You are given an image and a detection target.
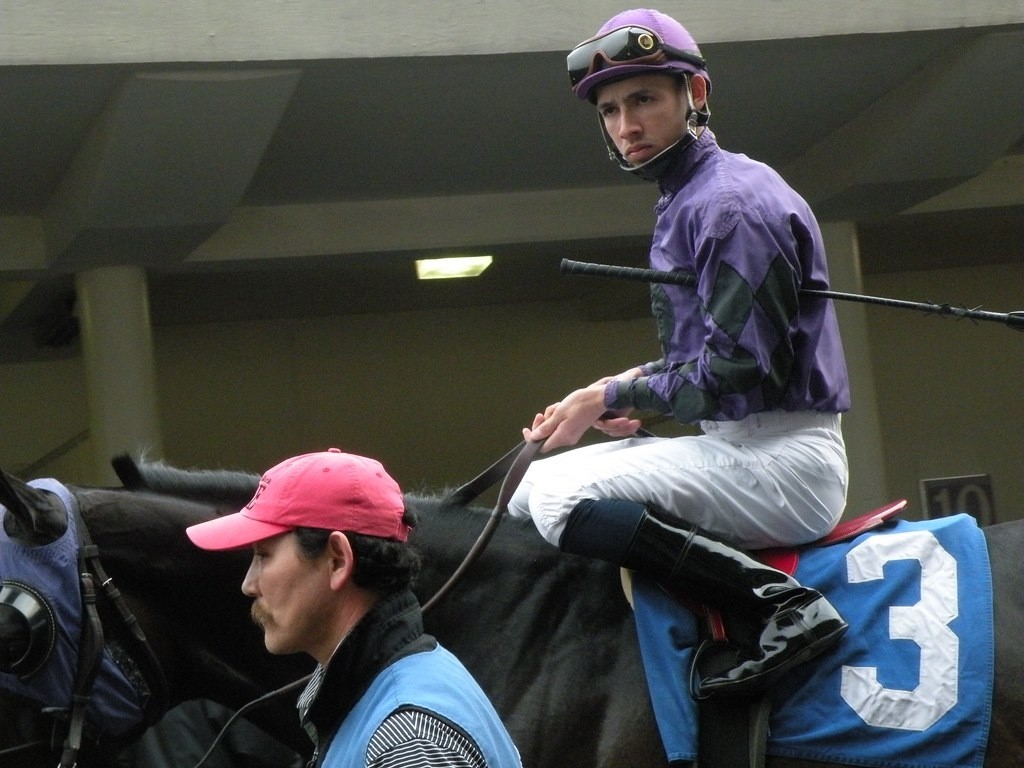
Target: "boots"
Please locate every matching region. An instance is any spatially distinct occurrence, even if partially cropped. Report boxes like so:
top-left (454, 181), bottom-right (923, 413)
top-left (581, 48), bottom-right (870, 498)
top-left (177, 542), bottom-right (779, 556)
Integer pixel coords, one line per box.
top-left (618, 501), bottom-right (849, 698)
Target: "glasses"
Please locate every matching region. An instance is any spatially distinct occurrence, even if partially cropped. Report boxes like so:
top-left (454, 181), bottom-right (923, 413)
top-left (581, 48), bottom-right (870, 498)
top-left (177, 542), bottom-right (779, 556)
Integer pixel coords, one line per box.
top-left (565, 24), bottom-right (707, 94)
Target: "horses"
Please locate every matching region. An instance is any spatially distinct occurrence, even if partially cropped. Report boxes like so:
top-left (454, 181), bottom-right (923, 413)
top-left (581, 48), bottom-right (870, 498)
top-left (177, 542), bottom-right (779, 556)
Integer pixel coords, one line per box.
top-left (0, 453), bottom-right (1024, 768)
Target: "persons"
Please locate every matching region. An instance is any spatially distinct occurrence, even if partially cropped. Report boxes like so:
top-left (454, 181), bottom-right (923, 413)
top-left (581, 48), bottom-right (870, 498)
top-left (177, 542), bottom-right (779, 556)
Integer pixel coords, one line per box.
top-left (185, 447), bottom-right (521, 768)
top-left (505, 7), bottom-right (850, 697)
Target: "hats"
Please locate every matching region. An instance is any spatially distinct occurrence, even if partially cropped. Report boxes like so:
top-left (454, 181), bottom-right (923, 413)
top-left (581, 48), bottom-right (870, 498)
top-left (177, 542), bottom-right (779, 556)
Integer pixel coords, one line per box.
top-left (186, 448), bottom-right (413, 550)
top-left (571, 7), bottom-right (713, 104)
